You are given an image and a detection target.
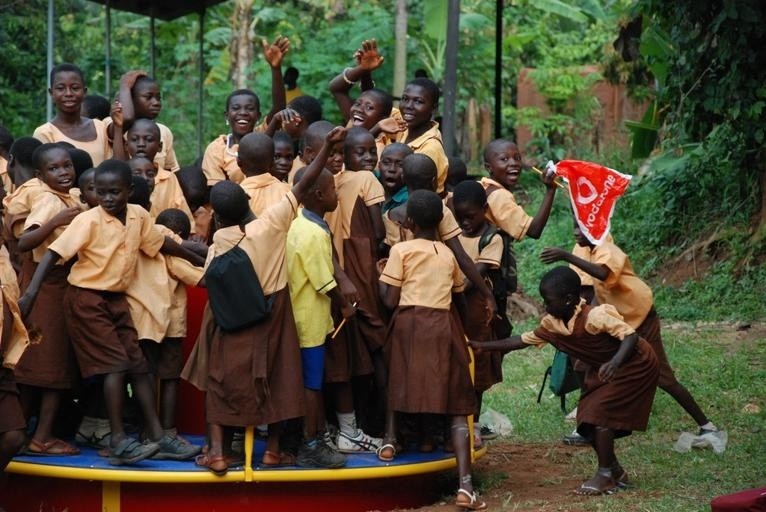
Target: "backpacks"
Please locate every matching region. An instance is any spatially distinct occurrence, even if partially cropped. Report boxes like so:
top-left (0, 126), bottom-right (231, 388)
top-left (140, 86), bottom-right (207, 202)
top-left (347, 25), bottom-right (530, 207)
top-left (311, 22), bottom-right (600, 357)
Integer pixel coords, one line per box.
top-left (536, 350), bottom-right (584, 416)
top-left (202, 235), bottom-right (276, 334)
top-left (478, 225), bottom-right (512, 333)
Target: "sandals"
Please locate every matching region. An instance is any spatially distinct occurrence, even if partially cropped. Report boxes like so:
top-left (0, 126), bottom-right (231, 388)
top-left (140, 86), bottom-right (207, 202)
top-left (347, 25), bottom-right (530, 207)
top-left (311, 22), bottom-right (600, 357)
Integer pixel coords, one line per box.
top-left (572, 467), bottom-right (628, 497)
top-left (90, 429), bottom-right (112, 448)
top-left (455, 487), bottom-right (488, 508)
top-left (375, 444), bottom-right (397, 461)
top-left (75, 432), bottom-right (97, 448)
top-left (195, 451), bottom-right (229, 474)
top-left (24, 437), bottom-right (81, 457)
top-left (258, 450), bottom-right (295, 469)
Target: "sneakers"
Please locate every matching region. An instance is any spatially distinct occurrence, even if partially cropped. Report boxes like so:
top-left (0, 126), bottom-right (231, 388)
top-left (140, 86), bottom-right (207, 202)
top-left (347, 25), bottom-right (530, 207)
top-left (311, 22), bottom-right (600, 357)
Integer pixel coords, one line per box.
top-left (478, 426), bottom-right (497, 440)
top-left (144, 433), bottom-right (202, 460)
top-left (563, 430), bottom-right (590, 446)
top-left (108, 438), bottom-right (159, 466)
top-left (303, 421), bottom-right (337, 450)
top-left (295, 440), bottom-right (346, 467)
top-left (336, 429), bottom-right (382, 454)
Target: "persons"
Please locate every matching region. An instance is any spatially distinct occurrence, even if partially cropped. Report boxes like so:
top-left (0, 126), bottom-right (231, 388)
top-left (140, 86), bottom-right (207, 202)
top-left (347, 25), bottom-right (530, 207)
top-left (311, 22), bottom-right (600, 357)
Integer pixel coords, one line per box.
top-left (539, 206), bottom-right (722, 450)
top-left (461, 263), bottom-right (665, 498)
top-left (194, 36), bottom-right (562, 510)
top-left (572, 225), bottom-right (617, 304)
top-left (0, 65), bottom-right (204, 465)
